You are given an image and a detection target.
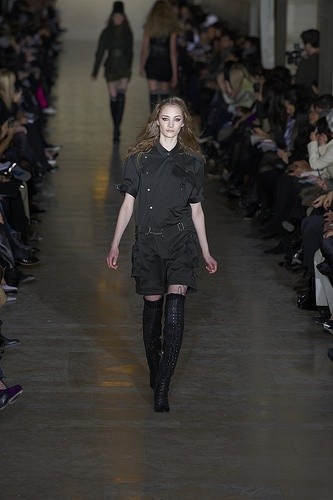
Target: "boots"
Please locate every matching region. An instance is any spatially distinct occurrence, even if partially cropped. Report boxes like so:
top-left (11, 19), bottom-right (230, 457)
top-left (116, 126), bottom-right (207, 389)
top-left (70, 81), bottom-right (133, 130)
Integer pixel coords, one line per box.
top-left (149, 89), bottom-right (159, 113)
top-left (160, 89), bottom-right (169, 102)
top-left (154, 294), bottom-right (185, 411)
top-left (110, 87), bottom-right (125, 142)
top-left (142, 295), bottom-right (165, 391)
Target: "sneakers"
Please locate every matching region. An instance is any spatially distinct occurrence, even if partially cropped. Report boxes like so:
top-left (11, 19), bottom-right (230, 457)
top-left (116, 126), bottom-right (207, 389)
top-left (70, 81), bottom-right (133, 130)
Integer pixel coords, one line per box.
top-left (0, 380), bottom-right (23, 411)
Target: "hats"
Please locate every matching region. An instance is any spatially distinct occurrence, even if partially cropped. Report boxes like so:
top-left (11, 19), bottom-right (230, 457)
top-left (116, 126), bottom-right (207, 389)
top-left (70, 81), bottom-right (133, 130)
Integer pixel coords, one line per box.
top-left (199, 14), bottom-right (219, 28)
top-left (264, 66), bottom-right (296, 81)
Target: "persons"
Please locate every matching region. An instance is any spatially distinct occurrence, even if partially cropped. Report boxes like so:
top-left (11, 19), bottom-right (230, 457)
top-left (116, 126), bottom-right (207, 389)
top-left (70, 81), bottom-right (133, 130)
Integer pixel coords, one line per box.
top-left (106, 96), bottom-right (217, 412)
top-left (140, 0), bottom-right (263, 179)
top-left (0, 0), bottom-right (70, 414)
top-left (90, 2), bottom-right (134, 142)
top-left (223, 0), bottom-right (333, 363)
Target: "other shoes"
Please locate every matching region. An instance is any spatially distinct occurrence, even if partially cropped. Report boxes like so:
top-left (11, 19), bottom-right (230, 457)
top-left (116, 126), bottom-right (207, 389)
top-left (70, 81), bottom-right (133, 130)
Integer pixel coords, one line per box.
top-left (207, 167), bottom-right (333, 337)
top-left (16, 270), bottom-right (35, 282)
top-left (6, 296), bottom-right (16, 301)
top-left (328, 348), bottom-right (333, 360)
top-left (1, 268), bottom-right (19, 290)
top-left (0, 105), bottom-right (64, 266)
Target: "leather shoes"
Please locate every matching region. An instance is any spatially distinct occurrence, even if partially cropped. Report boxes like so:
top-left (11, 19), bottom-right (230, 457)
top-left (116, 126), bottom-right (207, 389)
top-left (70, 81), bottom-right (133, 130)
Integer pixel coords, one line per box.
top-left (3, 339), bottom-right (20, 346)
